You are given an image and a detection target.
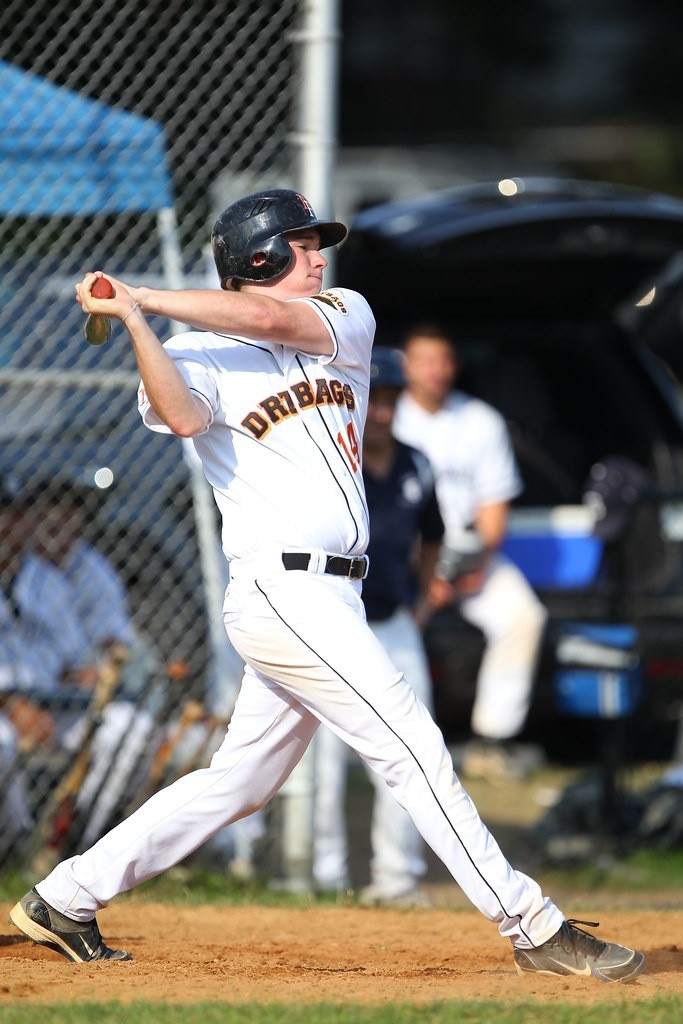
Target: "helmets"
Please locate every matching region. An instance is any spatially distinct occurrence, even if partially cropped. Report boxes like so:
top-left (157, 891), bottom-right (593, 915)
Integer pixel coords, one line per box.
top-left (211, 189), bottom-right (348, 294)
top-left (369, 347), bottom-right (406, 387)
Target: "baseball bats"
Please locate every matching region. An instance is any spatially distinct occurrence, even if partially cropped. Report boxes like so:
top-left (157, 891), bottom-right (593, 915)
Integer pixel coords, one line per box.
top-left (81, 278), bottom-right (113, 345)
top-left (1, 646), bottom-right (221, 877)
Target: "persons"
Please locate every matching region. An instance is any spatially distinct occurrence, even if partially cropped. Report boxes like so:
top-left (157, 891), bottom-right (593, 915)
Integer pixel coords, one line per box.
top-left (0, 186), bottom-right (650, 987)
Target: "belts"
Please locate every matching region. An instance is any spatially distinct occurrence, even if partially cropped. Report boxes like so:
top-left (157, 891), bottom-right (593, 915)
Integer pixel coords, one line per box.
top-left (281, 552), bottom-right (370, 579)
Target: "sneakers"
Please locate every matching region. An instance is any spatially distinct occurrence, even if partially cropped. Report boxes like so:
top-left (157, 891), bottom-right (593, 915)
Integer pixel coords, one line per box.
top-left (513, 919), bottom-right (645, 986)
top-left (9, 885), bottom-right (132, 967)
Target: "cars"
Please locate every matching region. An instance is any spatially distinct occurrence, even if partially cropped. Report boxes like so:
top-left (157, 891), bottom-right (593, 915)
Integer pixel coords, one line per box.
top-left (2, 172), bottom-right (681, 762)
